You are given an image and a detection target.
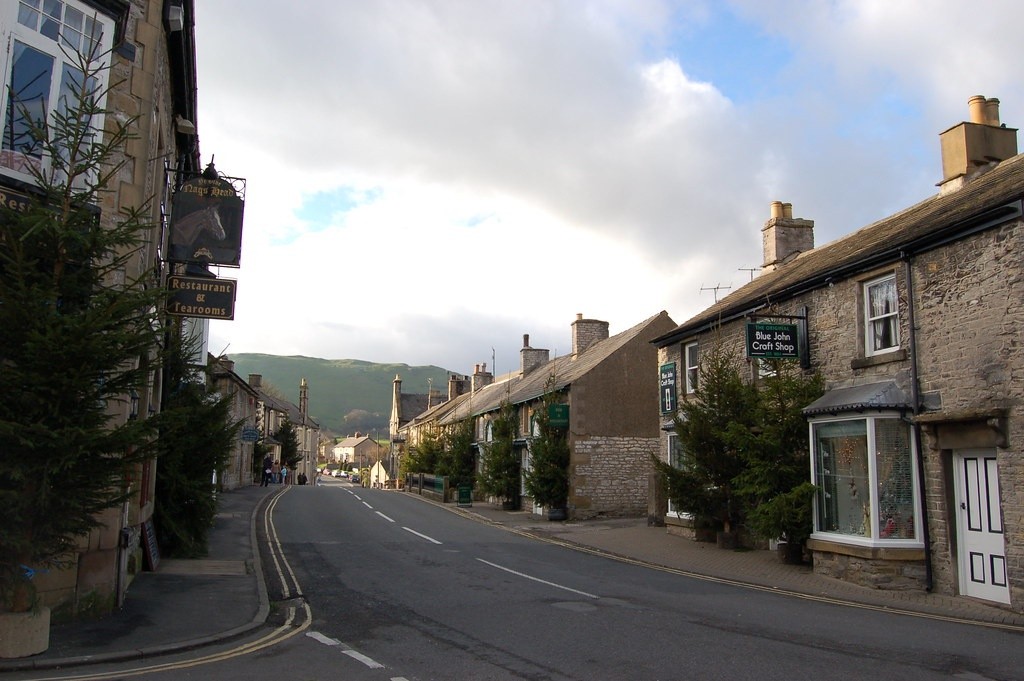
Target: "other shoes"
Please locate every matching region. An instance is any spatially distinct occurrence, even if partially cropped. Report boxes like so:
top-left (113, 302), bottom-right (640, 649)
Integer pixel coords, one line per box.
top-left (260, 484), bottom-right (262, 487)
top-left (265, 485), bottom-right (267, 487)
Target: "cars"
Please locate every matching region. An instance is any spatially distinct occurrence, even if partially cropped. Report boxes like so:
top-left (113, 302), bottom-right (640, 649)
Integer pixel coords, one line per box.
top-left (317, 467), bottom-right (360, 484)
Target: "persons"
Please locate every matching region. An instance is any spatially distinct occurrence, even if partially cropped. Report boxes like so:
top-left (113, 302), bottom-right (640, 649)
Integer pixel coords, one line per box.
top-left (260, 457), bottom-right (273, 487)
top-left (297, 472), bottom-right (307, 485)
top-left (272, 459), bottom-right (281, 484)
top-left (281, 463), bottom-right (287, 483)
